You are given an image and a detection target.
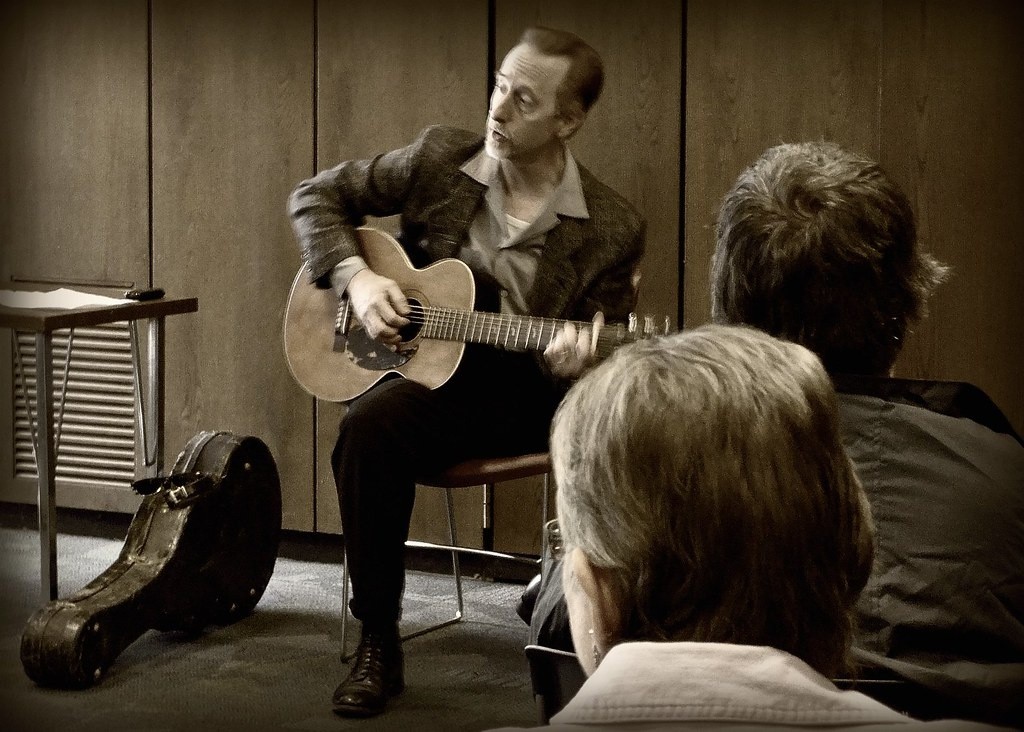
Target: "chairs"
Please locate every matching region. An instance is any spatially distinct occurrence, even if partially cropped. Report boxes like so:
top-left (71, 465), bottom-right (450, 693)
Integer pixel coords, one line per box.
top-left (340, 270), bottom-right (643, 664)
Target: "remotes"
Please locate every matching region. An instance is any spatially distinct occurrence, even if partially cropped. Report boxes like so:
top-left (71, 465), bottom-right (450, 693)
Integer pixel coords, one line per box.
top-left (123, 288), bottom-right (165, 302)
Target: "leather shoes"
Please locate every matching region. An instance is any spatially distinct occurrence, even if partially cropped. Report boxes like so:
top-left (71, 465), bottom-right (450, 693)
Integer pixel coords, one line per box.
top-left (332, 621), bottom-right (405, 713)
top-left (516, 574), bottom-right (541, 623)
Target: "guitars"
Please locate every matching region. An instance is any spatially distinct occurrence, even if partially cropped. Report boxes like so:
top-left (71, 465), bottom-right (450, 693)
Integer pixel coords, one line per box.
top-left (280, 227), bottom-right (679, 404)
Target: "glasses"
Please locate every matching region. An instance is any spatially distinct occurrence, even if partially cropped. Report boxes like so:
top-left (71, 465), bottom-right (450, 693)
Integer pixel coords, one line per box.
top-left (542, 516), bottom-right (580, 561)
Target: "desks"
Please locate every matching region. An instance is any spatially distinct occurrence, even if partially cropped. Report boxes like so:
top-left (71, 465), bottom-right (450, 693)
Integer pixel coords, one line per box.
top-left (0, 288), bottom-right (199, 608)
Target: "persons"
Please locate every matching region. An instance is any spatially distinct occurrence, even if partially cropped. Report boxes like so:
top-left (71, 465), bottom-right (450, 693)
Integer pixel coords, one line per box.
top-left (483, 139), bottom-right (1024, 732)
top-left (282, 25), bottom-right (645, 713)
